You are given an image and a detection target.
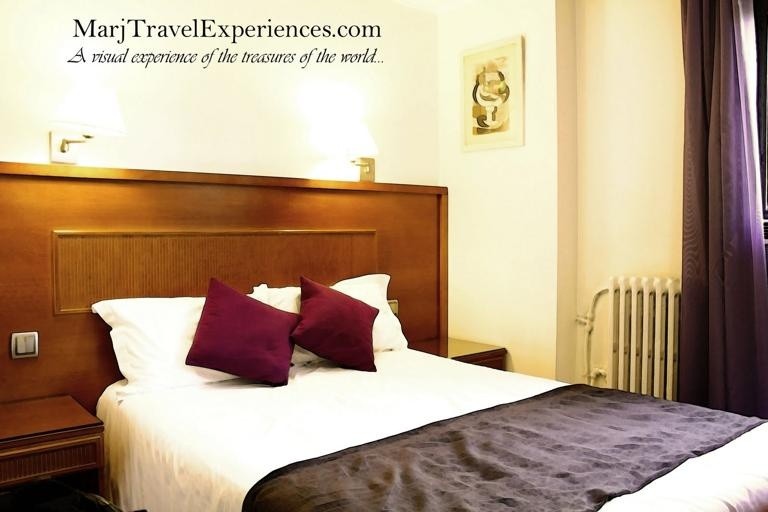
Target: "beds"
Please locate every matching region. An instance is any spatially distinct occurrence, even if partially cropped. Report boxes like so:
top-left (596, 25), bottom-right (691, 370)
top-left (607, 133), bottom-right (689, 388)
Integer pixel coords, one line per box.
top-left (94, 346), bottom-right (766, 512)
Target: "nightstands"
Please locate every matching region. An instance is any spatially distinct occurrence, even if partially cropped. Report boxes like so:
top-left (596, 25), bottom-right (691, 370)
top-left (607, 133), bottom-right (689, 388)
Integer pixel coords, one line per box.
top-left (447, 335), bottom-right (510, 369)
top-left (1, 397), bottom-right (108, 511)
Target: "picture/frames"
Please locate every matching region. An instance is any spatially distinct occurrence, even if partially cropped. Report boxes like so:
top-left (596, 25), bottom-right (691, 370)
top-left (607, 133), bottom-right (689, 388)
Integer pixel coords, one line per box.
top-left (458, 38), bottom-right (528, 153)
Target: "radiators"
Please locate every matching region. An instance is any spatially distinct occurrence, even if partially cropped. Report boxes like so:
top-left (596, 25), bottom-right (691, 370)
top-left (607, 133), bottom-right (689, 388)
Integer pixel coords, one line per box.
top-left (577, 278), bottom-right (681, 404)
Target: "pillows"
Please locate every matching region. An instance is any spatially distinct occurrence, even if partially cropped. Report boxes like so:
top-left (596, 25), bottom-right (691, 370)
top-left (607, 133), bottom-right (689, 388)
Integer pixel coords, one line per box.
top-left (87, 273), bottom-right (411, 385)
